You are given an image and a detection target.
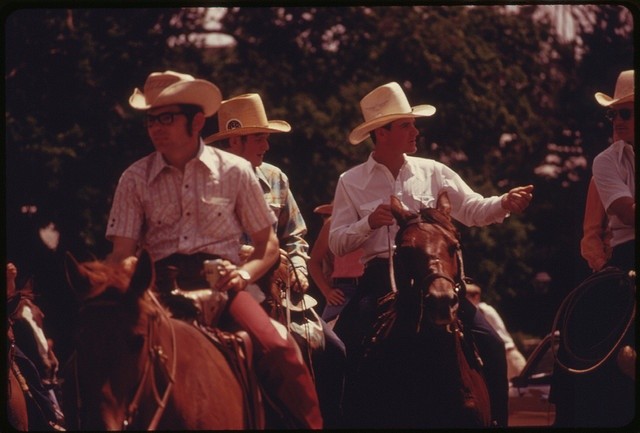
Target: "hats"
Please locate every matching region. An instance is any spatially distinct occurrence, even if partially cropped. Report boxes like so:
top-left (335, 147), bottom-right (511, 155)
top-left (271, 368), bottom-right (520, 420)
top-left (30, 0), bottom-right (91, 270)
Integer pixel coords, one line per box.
top-left (203, 92), bottom-right (291, 143)
top-left (348, 80), bottom-right (436, 144)
top-left (594, 69), bottom-right (635, 106)
top-left (129, 69), bottom-right (222, 119)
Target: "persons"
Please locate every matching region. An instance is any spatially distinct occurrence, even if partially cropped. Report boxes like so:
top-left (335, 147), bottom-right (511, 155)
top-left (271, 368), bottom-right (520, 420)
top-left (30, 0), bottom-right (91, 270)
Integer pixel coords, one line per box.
top-left (580, 121), bottom-right (622, 272)
top-left (310, 202), bottom-right (369, 323)
top-left (80, 69), bottom-right (330, 430)
top-left (539, 69), bottom-right (636, 429)
top-left (460, 283), bottom-right (529, 384)
top-left (327, 80), bottom-right (534, 427)
top-left (204, 90), bottom-right (349, 390)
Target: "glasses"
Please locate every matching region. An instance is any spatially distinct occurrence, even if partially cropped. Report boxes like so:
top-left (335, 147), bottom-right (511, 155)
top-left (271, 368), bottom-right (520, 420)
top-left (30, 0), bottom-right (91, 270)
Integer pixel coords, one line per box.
top-left (138, 108), bottom-right (193, 127)
top-left (605, 105), bottom-right (633, 121)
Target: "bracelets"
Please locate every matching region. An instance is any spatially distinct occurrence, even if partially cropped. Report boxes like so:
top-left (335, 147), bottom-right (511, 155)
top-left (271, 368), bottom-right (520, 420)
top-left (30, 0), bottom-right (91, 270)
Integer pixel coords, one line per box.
top-left (236, 267), bottom-right (252, 290)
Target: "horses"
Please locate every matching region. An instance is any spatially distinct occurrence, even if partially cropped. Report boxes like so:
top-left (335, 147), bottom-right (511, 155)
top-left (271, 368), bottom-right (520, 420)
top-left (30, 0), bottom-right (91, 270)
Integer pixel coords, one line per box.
top-left (552, 269), bottom-right (637, 426)
top-left (325, 189), bottom-right (497, 431)
top-left (6, 279), bottom-right (66, 432)
top-left (61, 250), bottom-right (280, 432)
